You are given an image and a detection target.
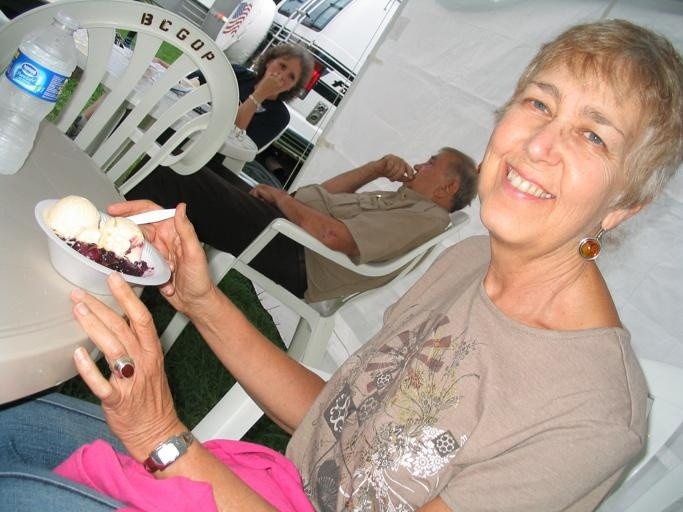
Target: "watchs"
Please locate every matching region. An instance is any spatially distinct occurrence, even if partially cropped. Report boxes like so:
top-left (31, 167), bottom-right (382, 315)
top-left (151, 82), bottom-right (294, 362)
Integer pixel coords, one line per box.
top-left (139, 429), bottom-right (195, 475)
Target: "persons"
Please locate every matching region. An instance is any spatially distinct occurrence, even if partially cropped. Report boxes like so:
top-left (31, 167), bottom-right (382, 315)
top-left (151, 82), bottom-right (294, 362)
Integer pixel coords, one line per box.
top-left (121, 145), bottom-right (478, 305)
top-left (0, 18), bottom-right (682, 511)
top-left (62, 43), bottom-right (315, 165)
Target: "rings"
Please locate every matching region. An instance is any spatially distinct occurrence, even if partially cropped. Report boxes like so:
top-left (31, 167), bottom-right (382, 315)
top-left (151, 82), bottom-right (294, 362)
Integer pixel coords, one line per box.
top-left (106, 357), bottom-right (135, 378)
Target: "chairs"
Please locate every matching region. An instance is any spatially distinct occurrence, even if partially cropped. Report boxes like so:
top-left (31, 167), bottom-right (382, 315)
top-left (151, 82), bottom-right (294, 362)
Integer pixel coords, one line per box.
top-left (1, 2), bottom-right (682, 508)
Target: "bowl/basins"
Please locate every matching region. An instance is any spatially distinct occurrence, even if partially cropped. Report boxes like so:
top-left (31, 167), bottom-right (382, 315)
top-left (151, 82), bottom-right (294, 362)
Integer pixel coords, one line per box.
top-left (33, 196), bottom-right (174, 298)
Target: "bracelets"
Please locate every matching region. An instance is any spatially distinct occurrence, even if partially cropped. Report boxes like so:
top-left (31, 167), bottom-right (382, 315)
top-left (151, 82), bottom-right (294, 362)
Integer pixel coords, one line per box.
top-left (247, 94), bottom-right (260, 108)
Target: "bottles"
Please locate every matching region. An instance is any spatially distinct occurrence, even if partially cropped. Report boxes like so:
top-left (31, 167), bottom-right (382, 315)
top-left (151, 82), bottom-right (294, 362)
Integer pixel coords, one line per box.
top-left (0, 9), bottom-right (78, 177)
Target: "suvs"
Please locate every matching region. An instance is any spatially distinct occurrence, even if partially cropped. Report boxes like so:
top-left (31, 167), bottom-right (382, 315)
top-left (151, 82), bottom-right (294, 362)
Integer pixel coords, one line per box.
top-left (214, 0), bottom-right (403, 167)
